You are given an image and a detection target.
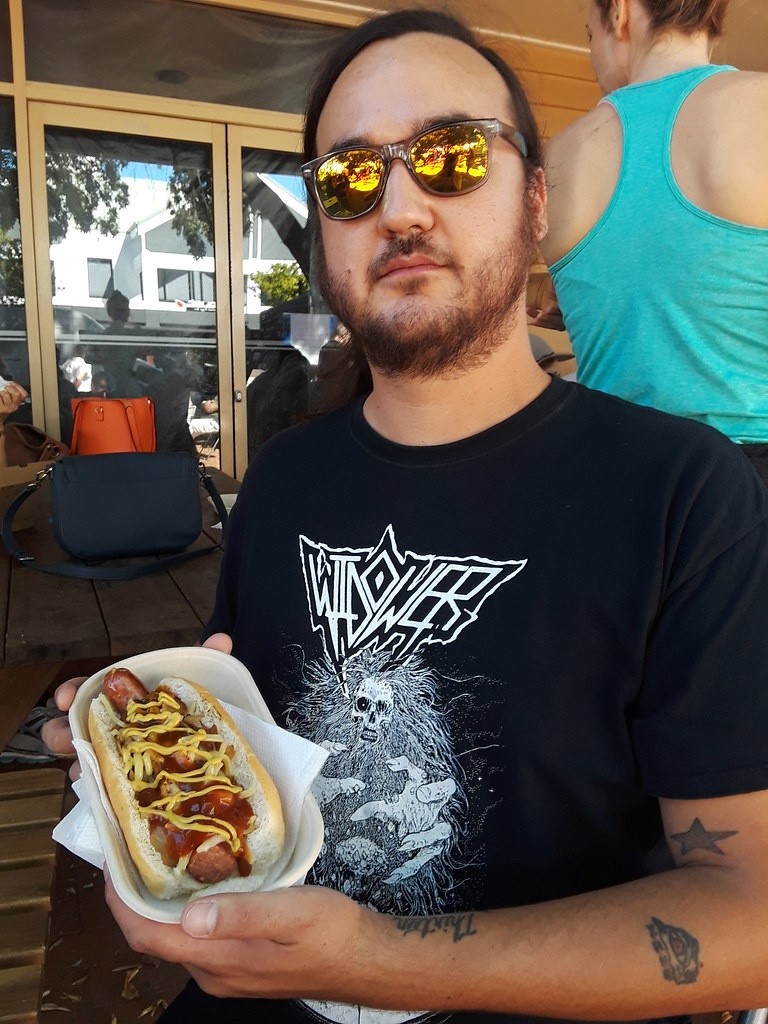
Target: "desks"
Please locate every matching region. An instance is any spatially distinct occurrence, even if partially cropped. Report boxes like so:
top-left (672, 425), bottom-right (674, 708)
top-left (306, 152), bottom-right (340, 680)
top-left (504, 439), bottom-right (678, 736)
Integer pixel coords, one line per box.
top-left (0, 467), bottom-right (242, 669)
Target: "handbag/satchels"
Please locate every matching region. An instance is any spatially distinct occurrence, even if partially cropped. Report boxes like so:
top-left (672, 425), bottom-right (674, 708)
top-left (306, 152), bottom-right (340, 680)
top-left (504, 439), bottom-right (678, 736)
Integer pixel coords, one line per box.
top-left (3, 452), bottom-right (228, 579)
top-left (70, 396), bottom-right (156, 452)
top-left (5, 423), bottom-right (68, 462)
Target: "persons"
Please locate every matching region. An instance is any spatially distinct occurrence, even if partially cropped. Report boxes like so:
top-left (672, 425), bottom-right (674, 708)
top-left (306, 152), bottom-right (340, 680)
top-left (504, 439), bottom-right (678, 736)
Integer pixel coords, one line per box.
top-left (79, 289), bottom-right (322, 452)
top-left (541, 1), bottom-right (766, 476)
top-left (0, 373), bottom-right (28, 470)
top-left (42, 10), bottom-right (766, 1023)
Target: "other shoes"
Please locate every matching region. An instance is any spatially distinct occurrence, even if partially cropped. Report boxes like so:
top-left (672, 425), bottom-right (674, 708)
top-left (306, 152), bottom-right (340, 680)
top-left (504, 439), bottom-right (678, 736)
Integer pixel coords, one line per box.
top-left (0, 698), bottom-right (67, 764)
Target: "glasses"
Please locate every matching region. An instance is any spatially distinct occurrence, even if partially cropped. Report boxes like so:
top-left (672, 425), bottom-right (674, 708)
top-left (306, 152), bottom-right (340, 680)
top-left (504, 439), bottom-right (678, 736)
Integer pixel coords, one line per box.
top-left (301, 117), bottom-right (528, 221)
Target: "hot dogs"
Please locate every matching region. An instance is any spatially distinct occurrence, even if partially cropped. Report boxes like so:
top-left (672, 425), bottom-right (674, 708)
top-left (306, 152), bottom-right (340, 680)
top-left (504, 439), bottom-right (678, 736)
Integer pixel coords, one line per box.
top-left (87, 667), bottom-right (284, 904)
